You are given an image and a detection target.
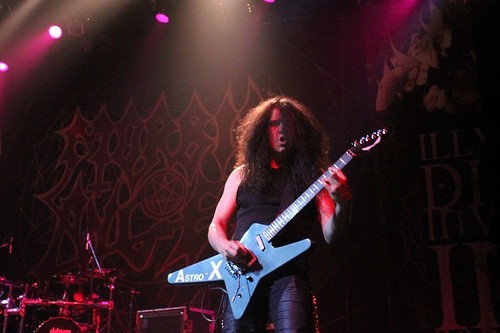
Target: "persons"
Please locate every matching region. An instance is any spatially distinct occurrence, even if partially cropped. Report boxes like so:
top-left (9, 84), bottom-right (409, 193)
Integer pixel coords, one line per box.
top-left (206, 95), bottom-right (352, 333)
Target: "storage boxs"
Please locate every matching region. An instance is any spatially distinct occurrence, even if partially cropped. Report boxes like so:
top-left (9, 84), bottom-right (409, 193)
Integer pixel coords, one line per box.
top-left (135, 306), bottom-right (216, 333)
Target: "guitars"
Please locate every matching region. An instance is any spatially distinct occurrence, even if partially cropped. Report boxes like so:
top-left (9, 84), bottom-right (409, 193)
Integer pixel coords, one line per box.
top-left (167, 127), bottom-right (388, 320)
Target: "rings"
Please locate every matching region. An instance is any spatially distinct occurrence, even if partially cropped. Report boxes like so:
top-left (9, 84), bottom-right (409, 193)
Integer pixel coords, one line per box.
top-left (335, 179), bottom-right (338, 183)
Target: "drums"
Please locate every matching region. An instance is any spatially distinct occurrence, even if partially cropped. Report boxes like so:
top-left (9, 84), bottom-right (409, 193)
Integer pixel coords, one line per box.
top-left (33, 316), bottom-right (83, 333)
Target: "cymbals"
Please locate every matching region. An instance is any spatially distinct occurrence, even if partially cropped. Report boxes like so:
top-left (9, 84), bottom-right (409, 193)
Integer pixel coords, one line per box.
top-left (51, 267), bottom-right (120, 284)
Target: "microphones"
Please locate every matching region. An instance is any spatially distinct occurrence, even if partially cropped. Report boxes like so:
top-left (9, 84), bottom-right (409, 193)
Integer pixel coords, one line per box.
top-left (9, 237), bottom-right (13, 253)
top-left (86, 233), bottom-right (89, 250)
